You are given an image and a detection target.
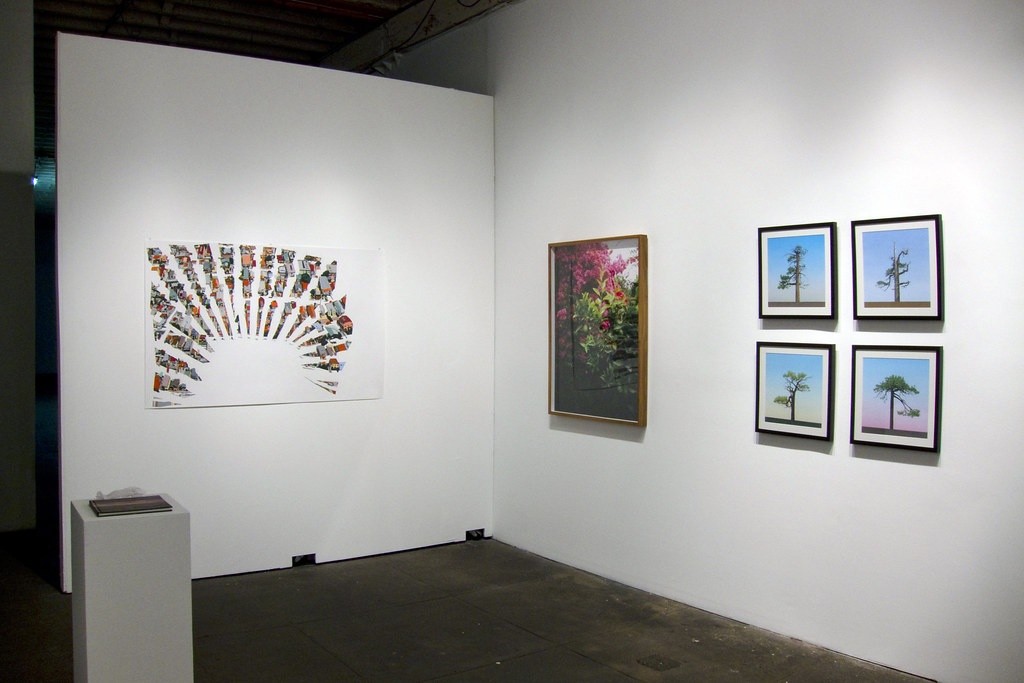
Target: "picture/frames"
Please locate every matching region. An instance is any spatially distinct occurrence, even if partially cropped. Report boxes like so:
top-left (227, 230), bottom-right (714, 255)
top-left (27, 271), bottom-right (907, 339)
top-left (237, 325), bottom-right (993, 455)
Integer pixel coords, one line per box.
top-left (852, 214), bottom-right (946, 321)
top-left (548, 234), bottom-right (648, 427)
top-left (755, 342), bottom-right (836, 441)
top-left (758, 222), bottom-right (839, 320)
top-left (850, 345), bottom-right (944, 453)
